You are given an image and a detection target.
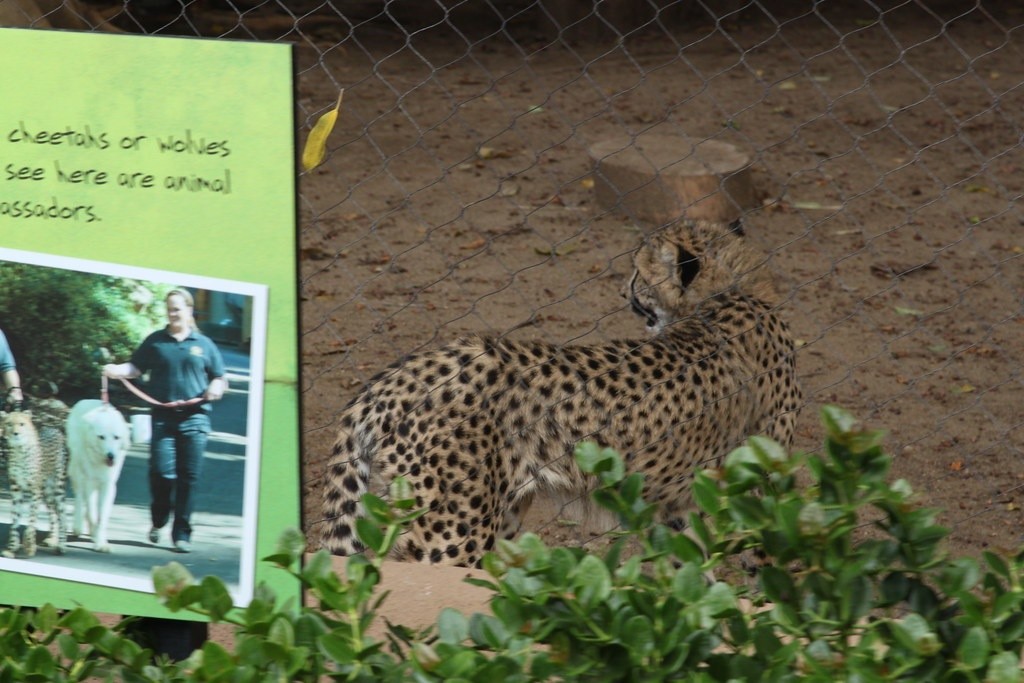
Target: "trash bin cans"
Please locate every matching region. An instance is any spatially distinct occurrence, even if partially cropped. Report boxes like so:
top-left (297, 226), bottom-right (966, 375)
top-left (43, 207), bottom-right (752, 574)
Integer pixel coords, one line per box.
top-left (128, 406), bottom-right (151, 444)
top-left (117, 615), bottom-right (209, 683)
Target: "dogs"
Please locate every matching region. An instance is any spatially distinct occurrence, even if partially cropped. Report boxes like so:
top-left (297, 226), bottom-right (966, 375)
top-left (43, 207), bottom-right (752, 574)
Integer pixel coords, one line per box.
top-left (0, 406), bottom-right (71, 557)
top-left (66, 397), bottom-right (134, 554)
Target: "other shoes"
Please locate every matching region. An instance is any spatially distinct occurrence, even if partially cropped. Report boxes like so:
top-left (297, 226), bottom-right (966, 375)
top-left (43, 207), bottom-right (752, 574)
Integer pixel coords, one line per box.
top-left (149, 526), bottom-right (161, 544)
top-left (175, 540), bottom-right (192, 553)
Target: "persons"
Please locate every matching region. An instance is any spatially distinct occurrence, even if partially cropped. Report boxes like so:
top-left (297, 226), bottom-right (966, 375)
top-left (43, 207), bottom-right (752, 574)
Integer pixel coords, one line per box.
top-left (103, 289), bottom-right (228, 553)
top-left (0, 330), bottom-right (24, 412)
